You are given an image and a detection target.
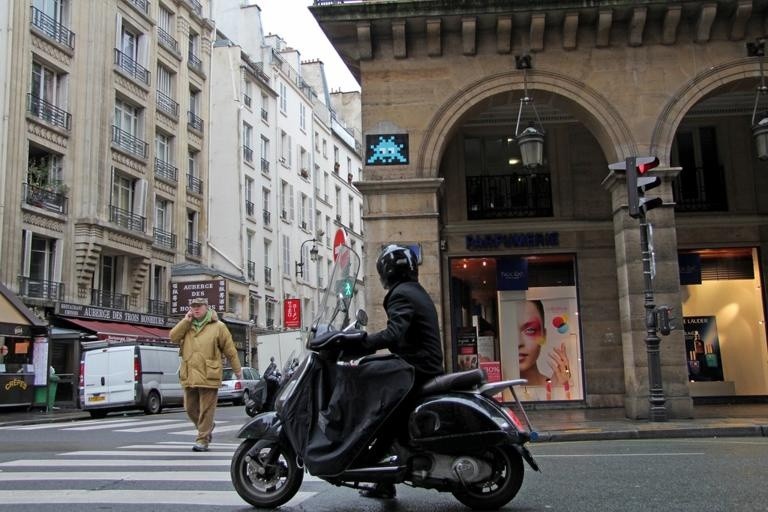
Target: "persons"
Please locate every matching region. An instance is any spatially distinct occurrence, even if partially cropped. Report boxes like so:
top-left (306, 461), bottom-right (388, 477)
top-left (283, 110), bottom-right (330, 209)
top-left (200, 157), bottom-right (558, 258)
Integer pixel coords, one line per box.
top-left (360, 243), bottom-right (445, 499)
top-left (169, 296), bottom-right (244, 452)
top-left (518, 299), bottom-right (571, 387)
top-left (0, 345), bottom-right (9, 363)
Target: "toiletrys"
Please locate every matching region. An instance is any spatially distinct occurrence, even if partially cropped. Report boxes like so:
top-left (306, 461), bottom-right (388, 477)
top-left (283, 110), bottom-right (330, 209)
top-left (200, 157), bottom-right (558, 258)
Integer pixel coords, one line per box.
top-left (459, 356), bottom-right (477, 370)
top-left (693, 330), bottom-right (705, 353)
top-left (706, 343), bottom-right (718, 368)
top-left (688, 350), bottom-right (701, 374)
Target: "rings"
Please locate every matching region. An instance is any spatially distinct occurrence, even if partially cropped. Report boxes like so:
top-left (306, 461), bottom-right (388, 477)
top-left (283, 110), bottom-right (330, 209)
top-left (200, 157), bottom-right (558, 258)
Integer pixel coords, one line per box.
top-left (566, 369), bottom-right (568, 373)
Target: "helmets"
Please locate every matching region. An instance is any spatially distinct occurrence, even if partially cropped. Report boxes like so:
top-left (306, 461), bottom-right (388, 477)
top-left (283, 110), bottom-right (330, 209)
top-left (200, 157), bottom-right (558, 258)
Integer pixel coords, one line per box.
top-left (376, 244), bottom-right (419, 290)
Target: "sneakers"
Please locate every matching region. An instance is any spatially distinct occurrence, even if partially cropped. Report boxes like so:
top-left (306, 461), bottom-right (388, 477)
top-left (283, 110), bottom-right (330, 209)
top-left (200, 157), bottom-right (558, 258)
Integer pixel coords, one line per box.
top-left (192, 422), bottom-right (216, 451)
top-left (358, 481), bottom-right (397, 498)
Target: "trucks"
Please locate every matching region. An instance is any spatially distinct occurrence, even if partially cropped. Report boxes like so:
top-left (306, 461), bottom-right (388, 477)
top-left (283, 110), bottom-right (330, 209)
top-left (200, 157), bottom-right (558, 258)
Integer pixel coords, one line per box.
top-left (79, 346), bottom-right (185, 416)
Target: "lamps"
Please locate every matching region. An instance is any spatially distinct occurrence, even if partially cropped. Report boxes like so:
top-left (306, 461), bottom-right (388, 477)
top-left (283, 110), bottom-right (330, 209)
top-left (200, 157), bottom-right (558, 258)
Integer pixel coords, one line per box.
top-left (512, 55), bottom-right (547, 170)
top-left (295, 238), bottom-right (318, 278)
top-left (744, 37), bottom-right (768, 161)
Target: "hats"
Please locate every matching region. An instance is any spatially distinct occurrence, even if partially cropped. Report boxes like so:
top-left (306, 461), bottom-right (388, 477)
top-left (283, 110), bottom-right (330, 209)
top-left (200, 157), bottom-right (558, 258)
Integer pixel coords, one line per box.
top-left (190, 297), bottom-right (209, 307)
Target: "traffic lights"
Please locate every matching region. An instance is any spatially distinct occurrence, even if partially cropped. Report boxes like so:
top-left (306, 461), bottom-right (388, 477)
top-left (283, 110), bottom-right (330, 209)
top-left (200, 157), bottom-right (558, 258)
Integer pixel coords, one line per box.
top-left (634, 157), bottom-right (663, 218)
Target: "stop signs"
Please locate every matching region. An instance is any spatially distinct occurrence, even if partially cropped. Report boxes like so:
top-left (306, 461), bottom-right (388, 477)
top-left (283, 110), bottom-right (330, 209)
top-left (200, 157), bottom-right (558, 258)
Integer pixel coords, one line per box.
top-left (333, 230), bottom-right (348, 268)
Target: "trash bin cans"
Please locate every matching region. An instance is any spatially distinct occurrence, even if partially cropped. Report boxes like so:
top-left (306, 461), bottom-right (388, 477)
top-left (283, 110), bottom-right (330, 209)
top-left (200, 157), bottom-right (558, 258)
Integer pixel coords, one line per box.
top-left (48, 374), bottom-right (60, 411)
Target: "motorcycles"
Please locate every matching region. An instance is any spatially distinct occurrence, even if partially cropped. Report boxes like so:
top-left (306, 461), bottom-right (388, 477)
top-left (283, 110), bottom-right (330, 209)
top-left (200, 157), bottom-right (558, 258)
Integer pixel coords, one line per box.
top-left (246, 348), bottom-right (306, 417)
top-left (231, 244), bottom-right (539, 510)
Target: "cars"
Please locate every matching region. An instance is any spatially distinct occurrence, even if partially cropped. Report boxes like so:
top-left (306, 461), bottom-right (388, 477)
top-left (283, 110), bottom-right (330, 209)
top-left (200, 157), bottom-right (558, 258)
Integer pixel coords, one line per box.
top-left (216, 366), bottom-right (262, 406)
